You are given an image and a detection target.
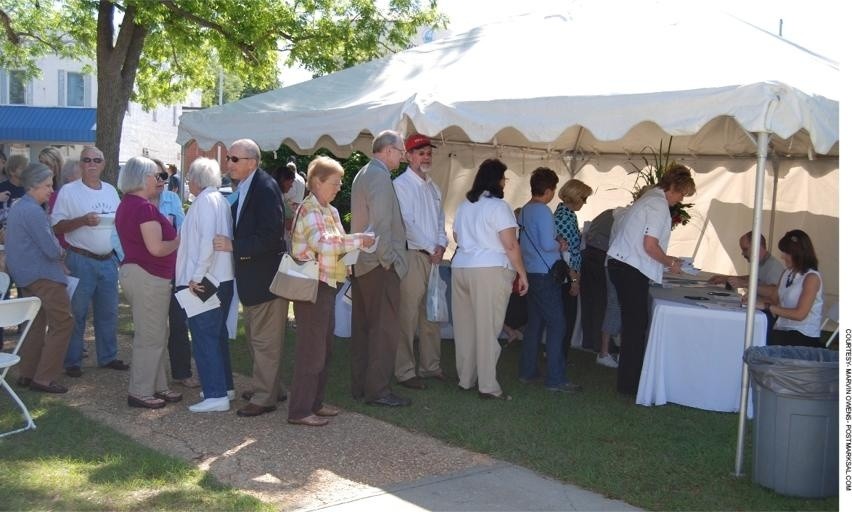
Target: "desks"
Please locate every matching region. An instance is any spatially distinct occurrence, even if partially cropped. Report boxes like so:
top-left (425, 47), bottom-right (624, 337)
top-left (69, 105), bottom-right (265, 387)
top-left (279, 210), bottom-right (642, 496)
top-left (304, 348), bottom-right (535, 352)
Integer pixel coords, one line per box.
top-left (635, 270), bottom-right (767, 421)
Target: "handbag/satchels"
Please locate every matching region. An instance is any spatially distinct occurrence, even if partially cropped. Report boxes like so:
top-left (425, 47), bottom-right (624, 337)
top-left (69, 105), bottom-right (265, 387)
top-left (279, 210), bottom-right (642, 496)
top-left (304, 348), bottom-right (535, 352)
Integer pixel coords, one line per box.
top-left (548, 259), bottom-right (571, 292)
top-left (268, 250), bottom-right (320, 304)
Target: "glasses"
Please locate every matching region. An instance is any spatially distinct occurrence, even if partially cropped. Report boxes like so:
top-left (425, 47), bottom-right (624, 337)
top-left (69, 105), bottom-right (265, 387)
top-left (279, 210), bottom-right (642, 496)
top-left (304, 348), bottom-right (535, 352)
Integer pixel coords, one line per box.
top-left (224, 153), bottom-right (253, 165)
top-left (152, 171), bottom-right (172, 182)
top-left (80, 155), bottom-right (106, 165)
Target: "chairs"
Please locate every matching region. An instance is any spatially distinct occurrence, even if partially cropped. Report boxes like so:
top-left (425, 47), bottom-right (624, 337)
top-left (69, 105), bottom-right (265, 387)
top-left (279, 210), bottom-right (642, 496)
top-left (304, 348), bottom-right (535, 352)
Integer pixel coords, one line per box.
top-left (0, 296), bottom-right (42, 440)
top-left (818, 314), bottom-right (838, 352)
top-left (0, 271), bottom-right (10, 302)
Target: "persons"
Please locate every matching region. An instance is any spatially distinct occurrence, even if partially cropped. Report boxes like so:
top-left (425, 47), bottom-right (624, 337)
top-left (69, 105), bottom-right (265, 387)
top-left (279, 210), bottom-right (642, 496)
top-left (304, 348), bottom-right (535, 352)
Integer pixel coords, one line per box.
top-left (739, 230), bottom-right (828, 349)
top-left (707, 231), bottom-right (784, 301)
top-left (450, 159), bottom-right (696, 399)
top-left (2, 146), bottom-right (198, 407)
top-left (173, 133), bottom-right (448, 426)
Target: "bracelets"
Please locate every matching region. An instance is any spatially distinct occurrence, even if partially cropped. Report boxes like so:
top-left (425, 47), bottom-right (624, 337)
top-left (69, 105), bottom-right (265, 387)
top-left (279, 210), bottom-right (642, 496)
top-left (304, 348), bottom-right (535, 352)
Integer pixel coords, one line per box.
top-left (765, 302), bottom-right (772, 311)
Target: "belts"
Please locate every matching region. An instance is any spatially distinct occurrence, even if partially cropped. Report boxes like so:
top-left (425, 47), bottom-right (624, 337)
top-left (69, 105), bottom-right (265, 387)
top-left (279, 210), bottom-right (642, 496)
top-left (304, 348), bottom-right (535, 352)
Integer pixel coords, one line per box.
top-left (67, 244), bottom-right (118, 262)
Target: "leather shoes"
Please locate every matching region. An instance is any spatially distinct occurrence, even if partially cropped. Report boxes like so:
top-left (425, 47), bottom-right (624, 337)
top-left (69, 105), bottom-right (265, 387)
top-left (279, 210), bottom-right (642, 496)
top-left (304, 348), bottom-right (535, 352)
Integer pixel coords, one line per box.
top-left (13, 344), bottom-right (623, 432)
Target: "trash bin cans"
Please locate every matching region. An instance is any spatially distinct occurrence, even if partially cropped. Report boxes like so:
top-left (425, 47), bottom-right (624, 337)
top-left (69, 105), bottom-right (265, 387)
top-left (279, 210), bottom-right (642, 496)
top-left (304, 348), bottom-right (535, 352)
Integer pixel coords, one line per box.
top-left (746, 345), bottom-right (838, 499)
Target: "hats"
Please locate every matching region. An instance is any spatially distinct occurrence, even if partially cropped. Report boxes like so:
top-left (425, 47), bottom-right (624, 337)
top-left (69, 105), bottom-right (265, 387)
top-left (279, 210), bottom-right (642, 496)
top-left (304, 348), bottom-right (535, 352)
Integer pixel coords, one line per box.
top-left (404, 134), bottom-right (437, 152)
